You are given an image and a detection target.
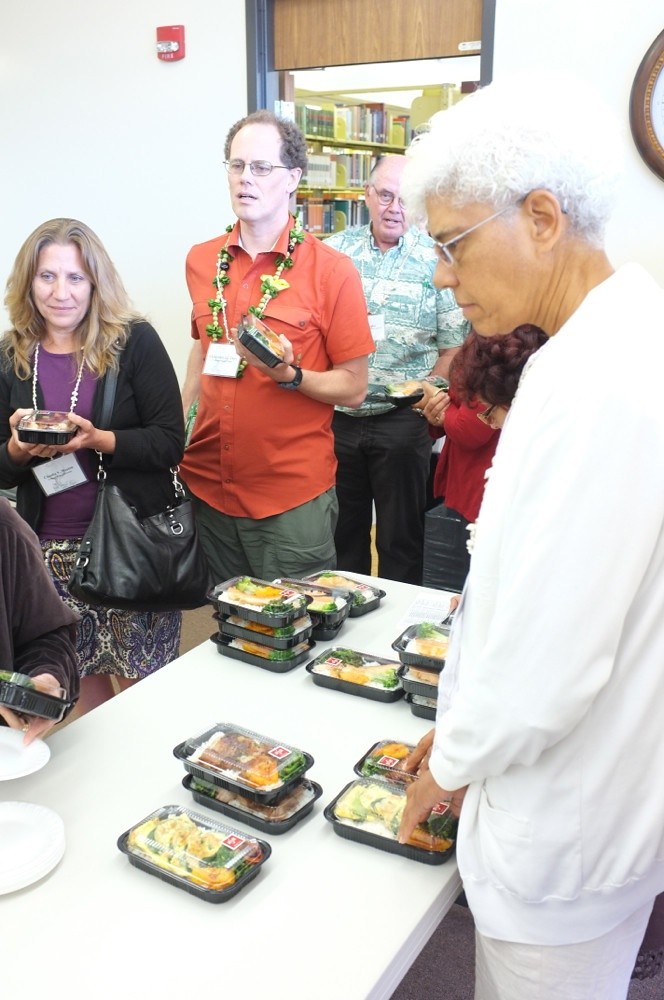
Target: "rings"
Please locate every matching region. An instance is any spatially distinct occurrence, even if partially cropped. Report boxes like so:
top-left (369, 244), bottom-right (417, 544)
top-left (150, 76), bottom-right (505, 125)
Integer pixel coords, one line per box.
top-left (23, 724), bottom-right (28, 732)
top-left (436, 417), bottom-right (440, 421)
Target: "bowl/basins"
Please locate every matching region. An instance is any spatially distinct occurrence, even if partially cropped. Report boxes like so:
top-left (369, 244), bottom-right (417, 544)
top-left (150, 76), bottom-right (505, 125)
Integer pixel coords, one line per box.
top-left (392, 623), bottom-right (451, 721)
top-left (323, 778), bottom-right (456, 865)
top-left (383, 375), bottom-right (450, 406)
top-left (237, 311), bottom-right (284, 369)
top-left (306, 647), bottom-right (407, 703)
top-left (204, 569), bottom-right (387, 674)
top-left (117, 805), bottom-right (272, 904)
top-left (0, 669), bottom-right (73, 723)
top-left (16, 410), bottom-right (79, 445)
top-left (173, 721), bottom-right (324, 835)
top-left (353, 739), bottom-right (428, 791)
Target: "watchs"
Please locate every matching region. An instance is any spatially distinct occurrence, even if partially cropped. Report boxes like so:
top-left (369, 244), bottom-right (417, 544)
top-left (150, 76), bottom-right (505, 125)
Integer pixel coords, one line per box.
top-left (477, 403), bottom-right (497, 423)
top-left (413, 408), bottom-right (423, 417)
top-left (278, 365), bottom-right (303, 390)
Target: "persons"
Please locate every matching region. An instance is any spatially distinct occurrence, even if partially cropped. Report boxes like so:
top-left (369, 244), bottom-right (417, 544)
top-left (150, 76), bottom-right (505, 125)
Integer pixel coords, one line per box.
top-left (412, 324), bottom-right (549, 524)
top-left (320, 154), bottom-right (470, 585)
top-left (184, 110), bottom-right (375, 586)
top-left (0, 217), bottom-right (186, 718)
top-left (0, 496), bottom-right (81, 744)
top-left (398, 73), bottom-right (663, 1000)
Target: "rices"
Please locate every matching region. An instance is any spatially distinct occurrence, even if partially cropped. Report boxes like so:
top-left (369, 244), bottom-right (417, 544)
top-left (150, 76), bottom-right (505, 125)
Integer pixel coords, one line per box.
top-left (404, 638), bottom-right (429, 706)
top-left (335, 596), bottom-right (348, 611)
top-left (216, 592), bottom-right (247, 652)
top-left (189, 779), bottom-right (314, 821)
top-left (292, 616), bottom-right (313, 656)
top-left (186, 731), bottom-right (284, 793)
top-left (311, 660), bottom-right (402, 691)
top-left (361, 590), bottom-right (379, 603)
top-left (335, 815), bottom-right (393, 838)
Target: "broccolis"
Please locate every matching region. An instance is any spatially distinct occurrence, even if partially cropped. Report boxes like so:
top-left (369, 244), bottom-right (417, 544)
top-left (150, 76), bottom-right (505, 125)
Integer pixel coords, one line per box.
top-left (318, 650), bottom-right (399, 688)
top-left (320, 602), bottom-right (338, 612)
top-left (235, 578), bottom-right (256, 592)
top-left (263, 600), bottom-right (297, 664)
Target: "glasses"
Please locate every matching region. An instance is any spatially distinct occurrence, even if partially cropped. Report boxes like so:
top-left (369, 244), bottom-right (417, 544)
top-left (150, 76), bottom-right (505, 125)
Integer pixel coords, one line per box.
top-left (430, 198), bottom-right (568, 269)
top-left (374, 184), bottom-right (405, 209)
top-left (223, 158), bottom-right (290, 176)
top-left (478, 403), bottom-right (498, 424)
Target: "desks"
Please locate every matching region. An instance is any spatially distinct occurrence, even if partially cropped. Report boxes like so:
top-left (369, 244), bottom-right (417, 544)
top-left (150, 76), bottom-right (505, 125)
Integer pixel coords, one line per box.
top-left (0, 568), bottom-right (467, 1000)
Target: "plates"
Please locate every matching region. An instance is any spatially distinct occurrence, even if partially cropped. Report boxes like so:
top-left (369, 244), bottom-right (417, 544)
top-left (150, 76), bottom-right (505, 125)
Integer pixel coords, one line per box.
top-left (0, 726), bottom-right (51, 781)
top-left (0, 800), bottom-right (68, 896)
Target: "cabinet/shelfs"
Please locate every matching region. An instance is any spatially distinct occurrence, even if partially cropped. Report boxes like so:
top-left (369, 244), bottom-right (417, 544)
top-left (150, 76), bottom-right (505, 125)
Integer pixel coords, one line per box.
top-left (290, 90), bottom-right (413, 240)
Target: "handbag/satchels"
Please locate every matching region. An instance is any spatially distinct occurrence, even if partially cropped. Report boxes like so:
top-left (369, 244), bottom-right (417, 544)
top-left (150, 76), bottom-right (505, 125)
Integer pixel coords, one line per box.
top-left (67, 479), bottom-right (209, 611)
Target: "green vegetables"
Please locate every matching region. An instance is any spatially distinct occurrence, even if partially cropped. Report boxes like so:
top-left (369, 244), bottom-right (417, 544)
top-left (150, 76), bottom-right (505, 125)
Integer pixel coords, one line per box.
top-left (192, 784), bottom-right (217, 799)
top-left (432, 379), bottom-right (449, 389)
top-left (245, 325), bottom-right (269, 346)
top-left (351, 590), bottom-right (366, 606)
top-left (276, 750), bottom-right (308, 780)
top-left (147, 828), bottom-right (255, 881)
top-left (423, 811), bottom-right (459, 838)
top-left (0, 671), bottom-right (35, 690)
top-left (361, 755), bottom-right (401, 777)
top-left (385, 384), bottom-right (394, 394)
top-left (321, 573), bottom-right (335, 577)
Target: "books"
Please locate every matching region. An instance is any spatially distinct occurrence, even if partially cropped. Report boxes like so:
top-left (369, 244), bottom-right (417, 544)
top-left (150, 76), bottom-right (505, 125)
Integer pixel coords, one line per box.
top-left (295, 103), bottom-right (417, 146)
top-left (295, 198), bottom-right (370, 234)
top-left (302, 147), bottom-right (390, 188)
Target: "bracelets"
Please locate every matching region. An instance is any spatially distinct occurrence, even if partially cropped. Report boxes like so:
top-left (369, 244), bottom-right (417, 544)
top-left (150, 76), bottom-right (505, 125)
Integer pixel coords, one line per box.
top-left (434, 388), bottom-right (448, 397)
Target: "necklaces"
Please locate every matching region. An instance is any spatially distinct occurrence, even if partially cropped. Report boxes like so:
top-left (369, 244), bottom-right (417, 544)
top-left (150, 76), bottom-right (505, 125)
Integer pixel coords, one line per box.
top-left (207, 212), bottom-right (304, 378)
